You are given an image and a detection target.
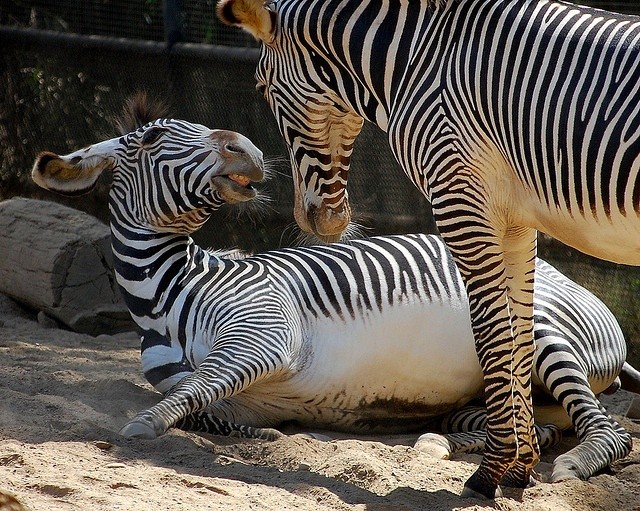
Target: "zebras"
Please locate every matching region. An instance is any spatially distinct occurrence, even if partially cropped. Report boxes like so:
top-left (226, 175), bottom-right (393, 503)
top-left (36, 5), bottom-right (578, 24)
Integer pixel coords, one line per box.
top-left (28, 89), bottom-right (639, 486)
top-left (214, 0), bottom-right (639, 505)
